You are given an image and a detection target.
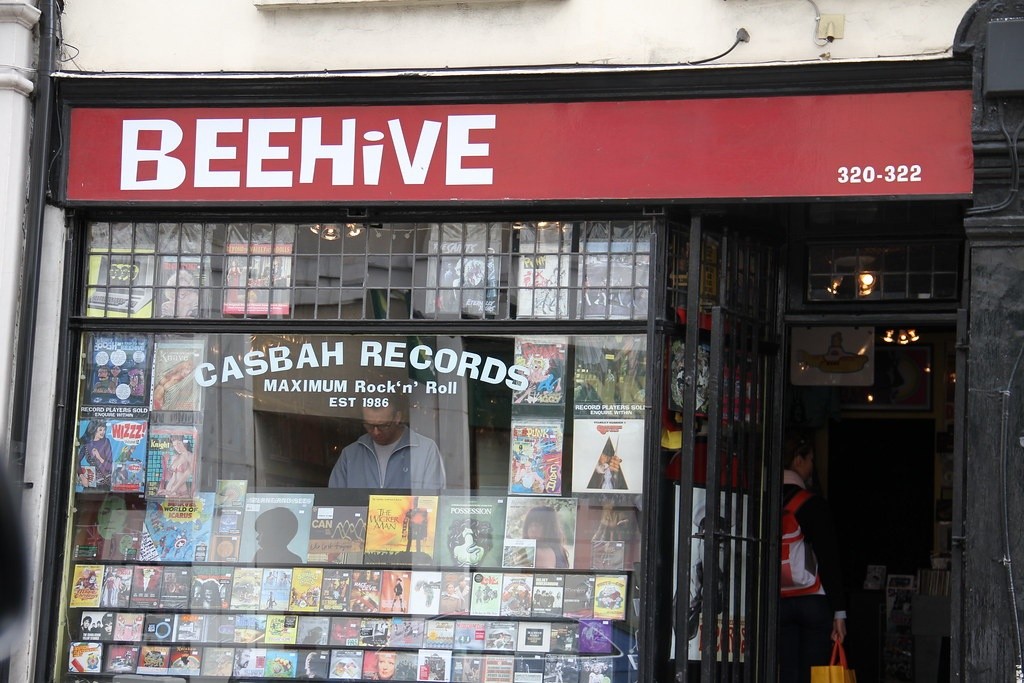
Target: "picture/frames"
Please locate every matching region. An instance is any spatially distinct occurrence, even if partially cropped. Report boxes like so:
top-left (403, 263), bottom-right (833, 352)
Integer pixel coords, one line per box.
top-left (788, 326), bottom-right (875, 387)
top-left (823, 344), bottom-right (934, 412)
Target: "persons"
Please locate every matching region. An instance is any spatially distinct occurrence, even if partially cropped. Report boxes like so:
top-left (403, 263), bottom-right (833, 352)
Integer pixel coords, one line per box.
top-left (250, 507), bottom-right (302, 563)
top-left (520, 505), bottom-right (570, 569)
top-left (362, 651), bottom-right (397, 679)
top-left (297, 652), bottom-right (325, 679)
top-left (774, 428), bottom-right (849, 683)
top-left (325, 386), bottom-right (446, 489)
top-left (192, 579), bottom-right (226, 607)
top-left (79, 613), bottom-right (114, 642)
top-left (442, 575), bottom-right (471, 612)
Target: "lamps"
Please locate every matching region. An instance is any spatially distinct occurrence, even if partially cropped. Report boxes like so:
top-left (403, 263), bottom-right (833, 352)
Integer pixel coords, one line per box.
top-left (826, 268), bottom-right (876, 296)
top-left (307, 223), bottom-right (366, 240)
top-left (512, 222), bottom-right (571, 234)
top-left (881, 329), bottom-right (920, 345)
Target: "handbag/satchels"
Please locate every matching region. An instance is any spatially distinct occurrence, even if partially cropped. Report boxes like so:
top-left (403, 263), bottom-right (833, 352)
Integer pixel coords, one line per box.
top-left (810, 640), bottom-right (858, 683)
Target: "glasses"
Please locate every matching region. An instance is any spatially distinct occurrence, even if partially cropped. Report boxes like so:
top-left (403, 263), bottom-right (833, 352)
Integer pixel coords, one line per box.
top-left (361, 416), bottom-right (396, 431)
